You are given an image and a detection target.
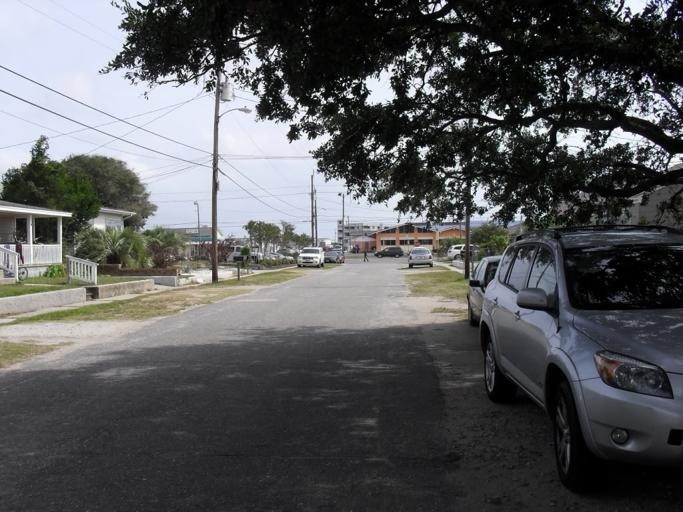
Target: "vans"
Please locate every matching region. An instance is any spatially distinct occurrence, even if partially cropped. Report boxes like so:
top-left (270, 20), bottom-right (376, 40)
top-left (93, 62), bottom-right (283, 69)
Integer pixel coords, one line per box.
top-left (447, 244), bottom-right (477, 260)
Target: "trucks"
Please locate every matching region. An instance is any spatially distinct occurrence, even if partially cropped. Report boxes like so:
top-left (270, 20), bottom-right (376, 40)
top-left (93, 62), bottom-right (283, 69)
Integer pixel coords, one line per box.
top-left (225, 246), bottom-right (260, 263)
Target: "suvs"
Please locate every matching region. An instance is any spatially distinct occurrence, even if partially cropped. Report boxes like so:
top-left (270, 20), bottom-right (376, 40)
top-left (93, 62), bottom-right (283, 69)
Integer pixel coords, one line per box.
top-left (477, 226), bottom-right (682, 492)
top-left (297, 247), bottom-right (324, 267)
top-left (373, 246), bottom-right (403, 258)
top-left (407, 246), bottom-right (432, 268)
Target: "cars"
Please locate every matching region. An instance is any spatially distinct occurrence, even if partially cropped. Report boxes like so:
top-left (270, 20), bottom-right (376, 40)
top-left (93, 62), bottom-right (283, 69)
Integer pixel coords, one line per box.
top-left (264, 249), bottom-right (295, 264)
top-left (466, 255), bottom-right (503, 325)
top-left (324, 245), bottom-right (344, 263)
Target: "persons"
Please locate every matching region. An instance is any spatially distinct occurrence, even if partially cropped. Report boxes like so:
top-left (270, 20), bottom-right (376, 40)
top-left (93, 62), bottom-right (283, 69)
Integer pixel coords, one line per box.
top-left (363, 249), bottom-right (368, 262)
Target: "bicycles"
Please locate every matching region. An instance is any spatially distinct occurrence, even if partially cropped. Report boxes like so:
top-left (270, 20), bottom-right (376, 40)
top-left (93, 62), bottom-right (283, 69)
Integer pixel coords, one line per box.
top-left (4, 265), bottom-right (28, 280)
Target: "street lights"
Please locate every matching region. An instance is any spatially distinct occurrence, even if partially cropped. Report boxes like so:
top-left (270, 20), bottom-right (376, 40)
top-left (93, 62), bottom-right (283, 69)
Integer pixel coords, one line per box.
top-left (211, 107), bottom-right (253, 284)
top-left (192, 200), bottom-right (200, 256)
